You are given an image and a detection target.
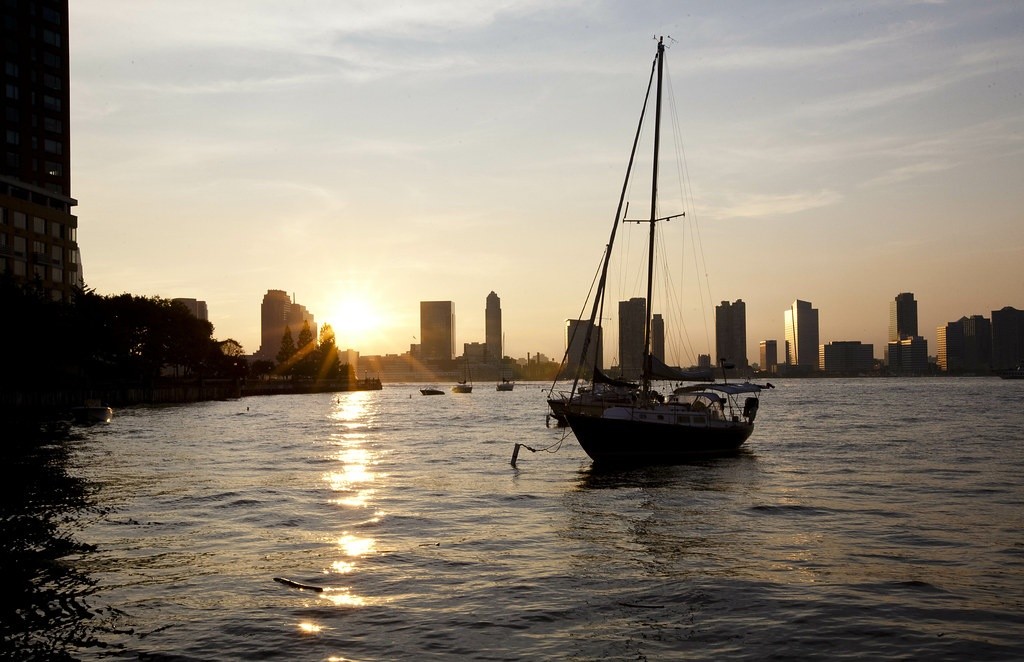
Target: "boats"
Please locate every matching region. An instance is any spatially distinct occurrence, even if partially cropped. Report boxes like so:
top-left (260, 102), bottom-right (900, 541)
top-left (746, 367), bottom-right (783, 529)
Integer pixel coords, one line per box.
top-left (357, 368), bottom-right (382, 390)
top-left (72, 406), bottom-right (114, 424)
top-left (451, 346), bottom-right (473, 392)
top-left (559, 32), bottom-right (762, 467)
top-left (998, 364), bottom-right (1024, 379)
top-left (419, 388), bottom-right (445, 395)
top-left (495, 334), bottom-right (513, 391)
top-left (542, 245), bottom-right (666, 421)
